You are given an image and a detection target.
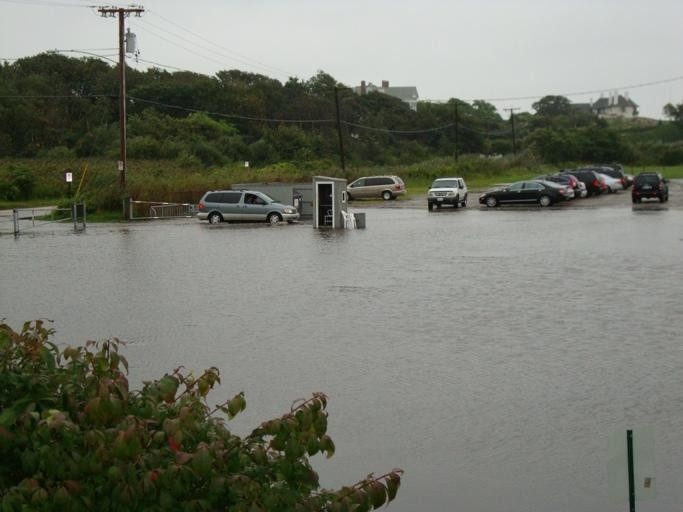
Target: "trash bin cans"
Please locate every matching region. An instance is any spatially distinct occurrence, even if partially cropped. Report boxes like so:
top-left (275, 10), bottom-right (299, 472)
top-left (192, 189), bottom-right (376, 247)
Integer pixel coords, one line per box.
top-left (354, 212), bottom-right (365, 229)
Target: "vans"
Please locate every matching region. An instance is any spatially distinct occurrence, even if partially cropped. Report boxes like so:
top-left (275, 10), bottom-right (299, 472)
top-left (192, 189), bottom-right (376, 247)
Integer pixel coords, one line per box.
top-left (344, 174), bottom-right (406, 201)
top-left (196, 188), bottom-right (300, 226)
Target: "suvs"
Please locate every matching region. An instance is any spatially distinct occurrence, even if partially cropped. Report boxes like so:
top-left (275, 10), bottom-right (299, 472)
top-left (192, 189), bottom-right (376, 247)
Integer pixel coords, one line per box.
top-left (425, 176), bottom-right (467, 210)
top-left (630, 171), bottom-right (668, 202)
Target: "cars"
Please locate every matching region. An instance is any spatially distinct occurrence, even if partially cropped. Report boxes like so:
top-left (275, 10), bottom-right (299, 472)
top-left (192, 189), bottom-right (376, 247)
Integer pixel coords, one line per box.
top-left (480, 162), bottom-right (636, 210)
